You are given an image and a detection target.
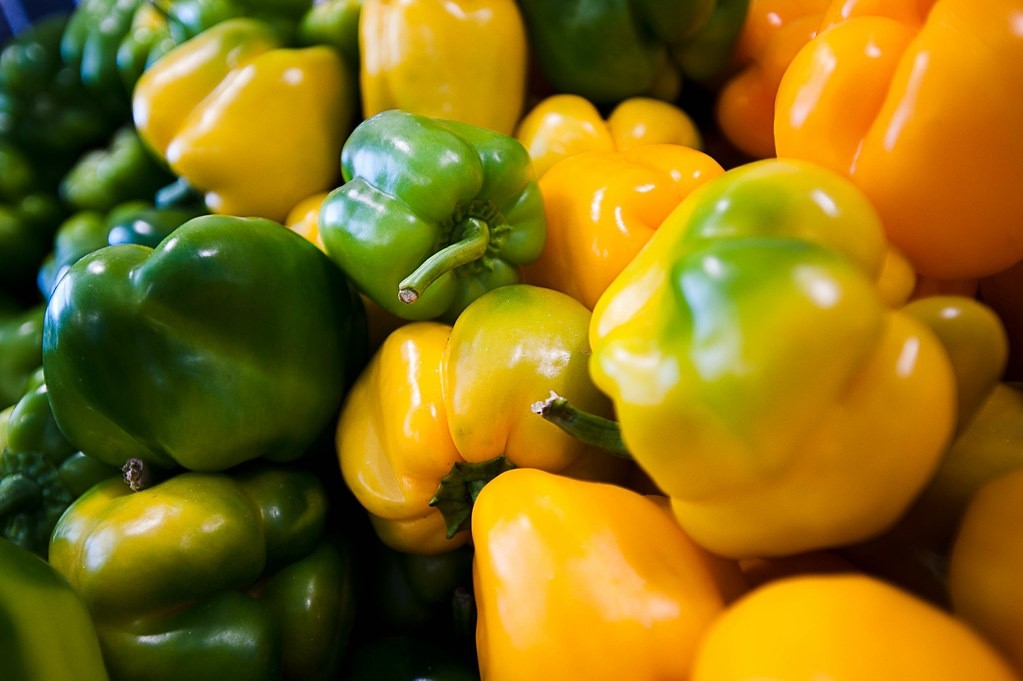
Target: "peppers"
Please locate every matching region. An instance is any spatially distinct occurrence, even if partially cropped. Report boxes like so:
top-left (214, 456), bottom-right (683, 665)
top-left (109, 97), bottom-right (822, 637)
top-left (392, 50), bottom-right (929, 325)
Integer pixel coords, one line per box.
top-left (0, 0), bottom-right (1023, 681)
